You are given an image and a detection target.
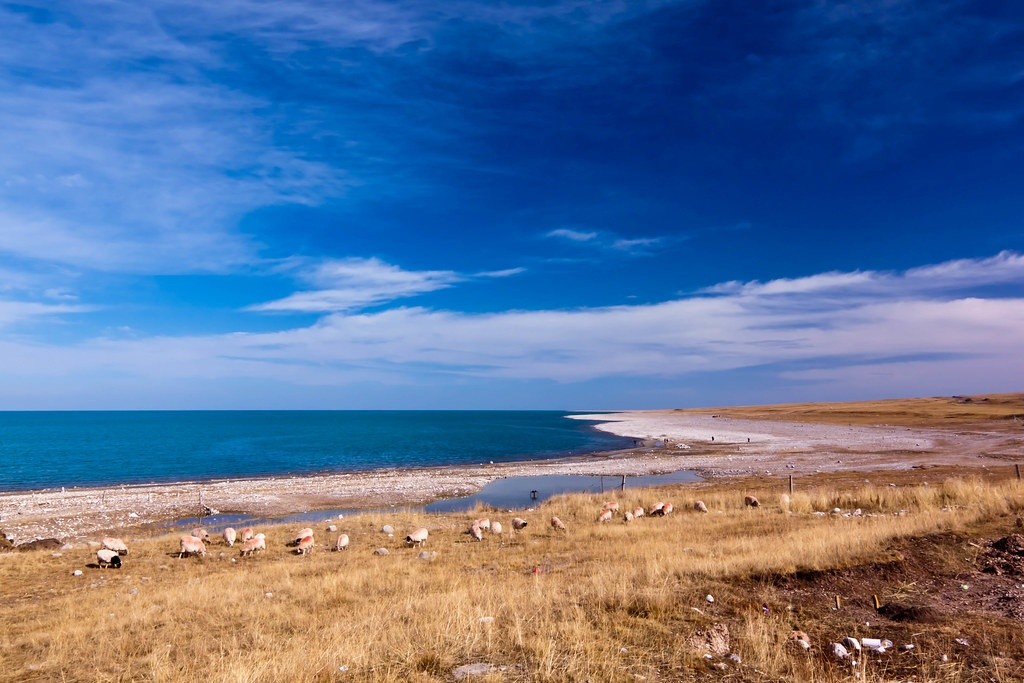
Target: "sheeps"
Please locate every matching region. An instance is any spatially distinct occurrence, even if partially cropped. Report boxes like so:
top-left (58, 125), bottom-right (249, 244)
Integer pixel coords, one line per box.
top-left (96, 495), bottom-right (761, 571)
